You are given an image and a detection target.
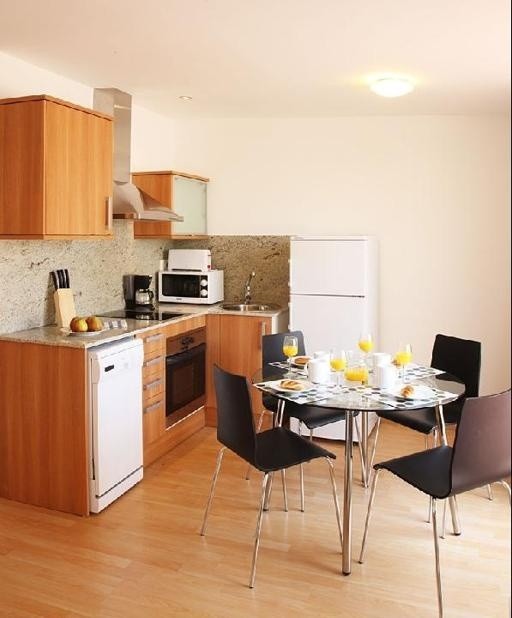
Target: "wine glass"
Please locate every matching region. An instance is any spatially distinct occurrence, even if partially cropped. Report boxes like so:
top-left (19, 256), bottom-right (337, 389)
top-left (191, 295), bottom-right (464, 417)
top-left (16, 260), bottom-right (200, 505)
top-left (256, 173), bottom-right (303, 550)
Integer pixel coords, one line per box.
top-left (283, 335), bottom-right (299, 378)
top-left (331, 332), bottom-right (414, 389)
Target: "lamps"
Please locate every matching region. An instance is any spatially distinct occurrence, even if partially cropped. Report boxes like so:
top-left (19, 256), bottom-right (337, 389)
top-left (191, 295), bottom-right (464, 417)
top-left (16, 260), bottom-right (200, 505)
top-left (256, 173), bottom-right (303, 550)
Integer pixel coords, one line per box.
top-left (366, 76), bottom-right (413, 99)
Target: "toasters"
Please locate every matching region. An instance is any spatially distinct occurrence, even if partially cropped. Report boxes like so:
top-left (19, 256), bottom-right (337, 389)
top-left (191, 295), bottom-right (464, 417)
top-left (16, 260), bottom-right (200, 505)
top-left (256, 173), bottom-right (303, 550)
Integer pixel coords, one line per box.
top-left (168, 248), bottom-right (212, 272)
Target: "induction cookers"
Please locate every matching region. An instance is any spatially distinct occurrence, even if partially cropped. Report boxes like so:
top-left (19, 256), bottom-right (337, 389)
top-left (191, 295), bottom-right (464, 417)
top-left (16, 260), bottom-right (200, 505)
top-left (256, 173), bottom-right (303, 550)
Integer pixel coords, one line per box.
top-left (95, 307), bottom-right (193, 321)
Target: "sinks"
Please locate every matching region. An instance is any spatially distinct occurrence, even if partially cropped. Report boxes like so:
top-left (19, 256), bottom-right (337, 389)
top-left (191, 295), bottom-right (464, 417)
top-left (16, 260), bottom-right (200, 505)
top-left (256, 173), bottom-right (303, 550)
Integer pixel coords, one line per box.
top-left (222, 303), bottom-right (267, 312)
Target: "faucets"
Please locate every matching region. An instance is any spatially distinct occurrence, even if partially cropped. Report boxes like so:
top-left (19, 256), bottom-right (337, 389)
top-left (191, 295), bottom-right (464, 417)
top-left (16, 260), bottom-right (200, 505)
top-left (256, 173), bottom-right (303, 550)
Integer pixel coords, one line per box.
top-left (242, 271), bottom-right (256, 304)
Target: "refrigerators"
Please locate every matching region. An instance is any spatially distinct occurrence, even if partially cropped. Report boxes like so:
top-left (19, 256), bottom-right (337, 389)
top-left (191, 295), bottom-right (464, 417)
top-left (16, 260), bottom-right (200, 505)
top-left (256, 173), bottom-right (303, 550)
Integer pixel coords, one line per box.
top-left (287, 233), bottom-right (383, 444)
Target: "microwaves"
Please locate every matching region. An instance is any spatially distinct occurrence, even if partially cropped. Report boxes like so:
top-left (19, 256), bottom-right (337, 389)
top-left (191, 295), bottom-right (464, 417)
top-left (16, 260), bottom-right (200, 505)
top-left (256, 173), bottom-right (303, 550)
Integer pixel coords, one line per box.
top-left (157, 269), bottom-right (225, 305)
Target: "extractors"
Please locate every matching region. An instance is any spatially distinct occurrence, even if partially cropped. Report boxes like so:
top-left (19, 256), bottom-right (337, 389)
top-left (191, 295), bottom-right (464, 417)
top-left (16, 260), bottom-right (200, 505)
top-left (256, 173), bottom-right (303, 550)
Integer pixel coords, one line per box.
top-left (92, 87), bottom-right (185, 222)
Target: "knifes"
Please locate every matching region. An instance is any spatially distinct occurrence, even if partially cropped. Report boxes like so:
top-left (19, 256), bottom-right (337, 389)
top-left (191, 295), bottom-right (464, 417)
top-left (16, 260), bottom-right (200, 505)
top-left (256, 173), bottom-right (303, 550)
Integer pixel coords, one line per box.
top-left (50, 267), bottom-right (71, 290)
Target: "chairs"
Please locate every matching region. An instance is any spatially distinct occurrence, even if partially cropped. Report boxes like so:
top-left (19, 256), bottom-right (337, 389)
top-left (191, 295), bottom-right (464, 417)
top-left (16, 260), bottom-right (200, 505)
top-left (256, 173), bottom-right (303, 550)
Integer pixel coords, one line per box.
top-left (256, 330), bottom-right (368, 516)
top-left (195, 362), bottom-right (347, 594)
top-left (357, 385), bottom-right (512, 618)
top-left (364, 327), bottom-right (496, 541)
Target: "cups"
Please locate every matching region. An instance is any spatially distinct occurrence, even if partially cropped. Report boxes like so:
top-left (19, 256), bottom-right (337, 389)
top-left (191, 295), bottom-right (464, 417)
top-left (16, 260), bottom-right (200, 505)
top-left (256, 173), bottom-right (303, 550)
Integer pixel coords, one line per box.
top-left (304, 359), bottom-right (330, 384)
top-left (313, 350), bottom-right (334, 369)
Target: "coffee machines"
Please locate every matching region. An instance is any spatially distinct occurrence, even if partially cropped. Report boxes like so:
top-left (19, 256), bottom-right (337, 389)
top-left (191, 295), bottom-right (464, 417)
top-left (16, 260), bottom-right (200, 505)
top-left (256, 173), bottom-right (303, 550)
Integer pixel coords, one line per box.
top-left (122, 273), bottom-right (156, 312)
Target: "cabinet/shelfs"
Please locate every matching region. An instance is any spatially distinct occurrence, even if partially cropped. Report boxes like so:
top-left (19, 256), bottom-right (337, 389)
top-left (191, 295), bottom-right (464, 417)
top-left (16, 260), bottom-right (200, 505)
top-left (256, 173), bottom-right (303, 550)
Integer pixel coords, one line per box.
top-left (1, 91), bottom-right (116, 243)
top-left (128, 170), bottom-right (212, 242)
top-left (203, 304), bottom-right (282, 429)
top-left (91, 302), bottom-right (165, 470)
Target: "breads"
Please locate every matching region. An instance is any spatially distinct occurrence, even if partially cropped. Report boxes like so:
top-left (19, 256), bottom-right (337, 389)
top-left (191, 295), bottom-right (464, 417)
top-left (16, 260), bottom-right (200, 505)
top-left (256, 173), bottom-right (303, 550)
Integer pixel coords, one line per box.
top-left (295, 357), bottom-right (310, 364)
top-left (401, 386), bottom-right (413, 398)
top-left (281, 380), bottom-right (302, 390)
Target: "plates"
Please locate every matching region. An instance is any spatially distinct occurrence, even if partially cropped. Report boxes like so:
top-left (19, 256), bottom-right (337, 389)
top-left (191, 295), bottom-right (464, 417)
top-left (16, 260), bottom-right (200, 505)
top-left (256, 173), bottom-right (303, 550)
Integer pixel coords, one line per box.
top-left (59, 328), bottom-right (106, 337)
top-left (286, 355), bottom-right (311, 367)
top-left (391, 386), bottom-right (433, 401)
top-left (271, 379), bottom-right (316, 393)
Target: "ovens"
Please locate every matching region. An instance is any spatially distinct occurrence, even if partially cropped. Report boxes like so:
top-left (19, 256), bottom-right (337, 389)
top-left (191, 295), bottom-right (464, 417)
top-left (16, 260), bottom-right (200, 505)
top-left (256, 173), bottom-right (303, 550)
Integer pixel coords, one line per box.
top-left (165, 325), bottom-right (207, 428)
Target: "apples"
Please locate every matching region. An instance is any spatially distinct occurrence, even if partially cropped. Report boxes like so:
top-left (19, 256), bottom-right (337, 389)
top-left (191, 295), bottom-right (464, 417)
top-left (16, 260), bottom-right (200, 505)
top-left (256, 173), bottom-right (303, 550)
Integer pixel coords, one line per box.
top-left (70, 315), bottom-right (102, 332)
